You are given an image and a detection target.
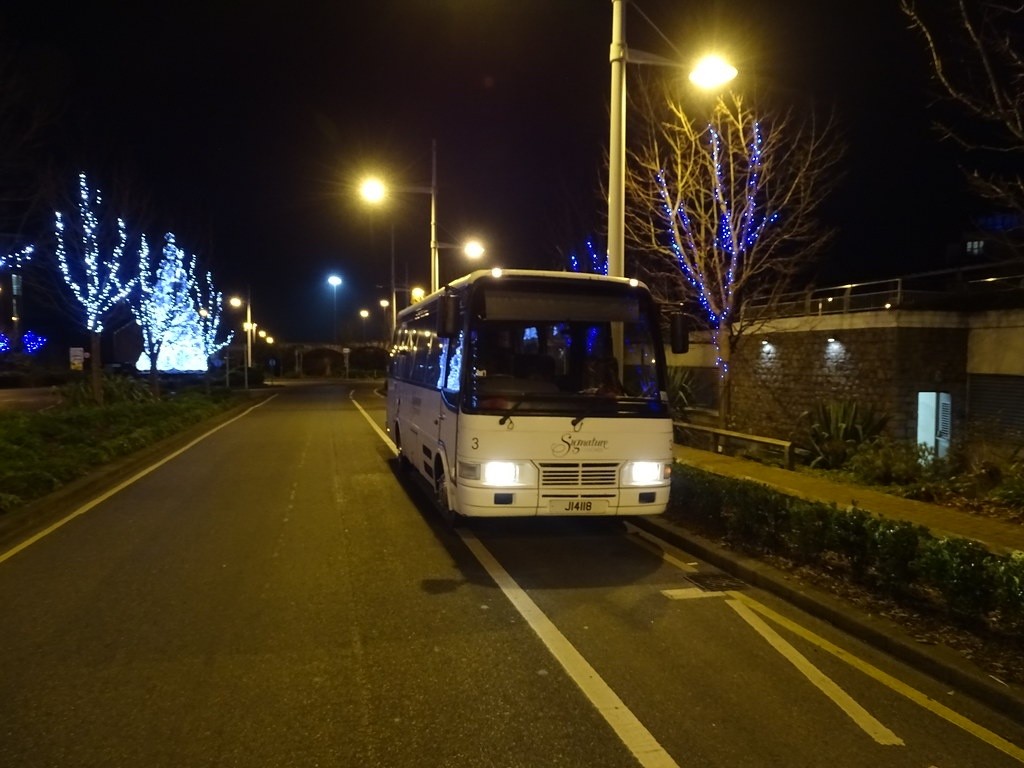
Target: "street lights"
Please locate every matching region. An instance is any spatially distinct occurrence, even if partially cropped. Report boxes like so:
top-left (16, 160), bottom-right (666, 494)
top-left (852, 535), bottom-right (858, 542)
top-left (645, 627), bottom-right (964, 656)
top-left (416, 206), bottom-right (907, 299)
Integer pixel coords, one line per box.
top-left (610, 0), bottom-right (739, 385)
top-left (328, 275), bottom-right (343, 339)
top-left (230, 287), bottom-right (251, 387)
top-left (357, 173), bottom-right (487, 296)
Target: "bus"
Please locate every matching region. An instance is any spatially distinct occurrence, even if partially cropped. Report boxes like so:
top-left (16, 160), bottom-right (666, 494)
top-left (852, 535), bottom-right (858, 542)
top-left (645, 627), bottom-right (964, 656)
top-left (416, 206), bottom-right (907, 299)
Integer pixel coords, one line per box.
top-left (382, 270), bottom-right (690, 523)
